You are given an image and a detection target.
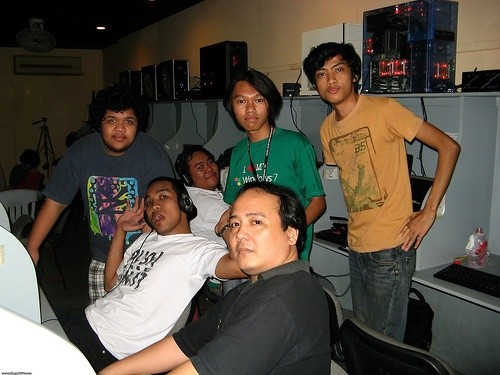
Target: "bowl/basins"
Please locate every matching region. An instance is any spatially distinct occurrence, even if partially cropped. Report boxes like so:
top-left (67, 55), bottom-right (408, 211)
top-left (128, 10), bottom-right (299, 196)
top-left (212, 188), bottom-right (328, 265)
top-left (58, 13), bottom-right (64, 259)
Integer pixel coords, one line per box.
top-left (467, 252), bottom-right (490, 269)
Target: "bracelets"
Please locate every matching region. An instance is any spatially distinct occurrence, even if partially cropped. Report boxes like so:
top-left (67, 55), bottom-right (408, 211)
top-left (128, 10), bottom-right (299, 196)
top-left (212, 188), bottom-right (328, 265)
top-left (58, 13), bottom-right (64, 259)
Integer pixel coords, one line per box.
top-left (219, 224), bottom-right (229, 236)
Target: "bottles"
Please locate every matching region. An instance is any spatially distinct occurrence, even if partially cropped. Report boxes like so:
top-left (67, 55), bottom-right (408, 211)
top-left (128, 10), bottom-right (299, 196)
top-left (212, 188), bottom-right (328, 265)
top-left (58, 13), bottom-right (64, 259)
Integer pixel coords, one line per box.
top-left (471, 226), bottom-right (487, 266)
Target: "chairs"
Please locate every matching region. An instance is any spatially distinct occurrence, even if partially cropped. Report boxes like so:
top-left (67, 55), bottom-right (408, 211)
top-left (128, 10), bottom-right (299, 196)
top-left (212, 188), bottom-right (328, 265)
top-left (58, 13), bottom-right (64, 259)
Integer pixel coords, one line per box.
top-left (0, 189), bottom-right (40, 224)
top-left (339, 319), bottom-right (455, 375)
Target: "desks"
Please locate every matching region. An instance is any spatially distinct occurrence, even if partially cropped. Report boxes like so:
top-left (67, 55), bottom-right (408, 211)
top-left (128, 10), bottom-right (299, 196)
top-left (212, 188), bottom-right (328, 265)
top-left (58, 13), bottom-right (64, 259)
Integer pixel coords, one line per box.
top-left (309, 233), bottom-right (500, 375)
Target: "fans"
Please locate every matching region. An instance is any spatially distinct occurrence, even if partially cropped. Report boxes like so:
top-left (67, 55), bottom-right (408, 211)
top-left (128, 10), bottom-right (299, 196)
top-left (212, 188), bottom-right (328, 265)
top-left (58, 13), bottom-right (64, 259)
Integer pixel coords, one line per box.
top-left (16, 19), bottom-right (56, 53)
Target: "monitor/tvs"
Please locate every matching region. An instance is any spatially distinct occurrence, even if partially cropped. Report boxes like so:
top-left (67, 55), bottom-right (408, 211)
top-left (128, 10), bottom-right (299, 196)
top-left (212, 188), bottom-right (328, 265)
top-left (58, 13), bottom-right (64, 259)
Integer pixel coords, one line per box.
top-left (410, 176), bottom-right (437, 211)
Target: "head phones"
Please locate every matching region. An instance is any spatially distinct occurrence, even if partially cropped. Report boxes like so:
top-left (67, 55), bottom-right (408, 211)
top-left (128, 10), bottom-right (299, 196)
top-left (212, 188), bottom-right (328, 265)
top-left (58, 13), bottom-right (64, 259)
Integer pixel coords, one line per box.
top-left (141, 180), bottom-right (194, 229)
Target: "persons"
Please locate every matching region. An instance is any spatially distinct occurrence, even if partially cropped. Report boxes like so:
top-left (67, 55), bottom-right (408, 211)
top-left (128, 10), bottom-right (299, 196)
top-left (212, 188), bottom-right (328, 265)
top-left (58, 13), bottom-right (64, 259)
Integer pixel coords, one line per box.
top-left (65, 176), bottom-right (250, 375)
top-left (177, 143), bottom-right (230, 248)
top-left (26, 84), bottom-right (175, 303)
top-left (97, 180), bottom-right (330, 375)
top-left (222, 67), bottom-right (327, 261)
top-left (303, 41), bottom-right (461, 343)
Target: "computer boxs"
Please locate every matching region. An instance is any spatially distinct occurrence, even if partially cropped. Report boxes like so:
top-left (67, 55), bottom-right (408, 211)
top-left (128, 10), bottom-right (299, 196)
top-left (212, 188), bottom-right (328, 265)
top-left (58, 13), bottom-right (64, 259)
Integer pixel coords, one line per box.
top-left (157, 57), bottom-right (189, 100)
top-left (119, 64), bottom-right (157, 100)
top-left (198, 40), bottom-right (249, 95)
top-left (362, 0), bottom-right (458, 94)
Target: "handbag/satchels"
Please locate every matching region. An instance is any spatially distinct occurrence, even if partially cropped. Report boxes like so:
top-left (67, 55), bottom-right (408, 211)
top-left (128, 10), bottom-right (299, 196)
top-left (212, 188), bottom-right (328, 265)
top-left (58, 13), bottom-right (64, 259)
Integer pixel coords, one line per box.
top-left (403, 288), bottom-right (434, 352)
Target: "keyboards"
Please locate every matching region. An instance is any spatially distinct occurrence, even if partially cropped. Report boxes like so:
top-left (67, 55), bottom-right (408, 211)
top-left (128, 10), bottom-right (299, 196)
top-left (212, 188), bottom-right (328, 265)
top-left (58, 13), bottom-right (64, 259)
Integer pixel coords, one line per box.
top-left (315, 227), bottom-right (347, 246)
top-left (432, 263), bottom-right (500, 298)
